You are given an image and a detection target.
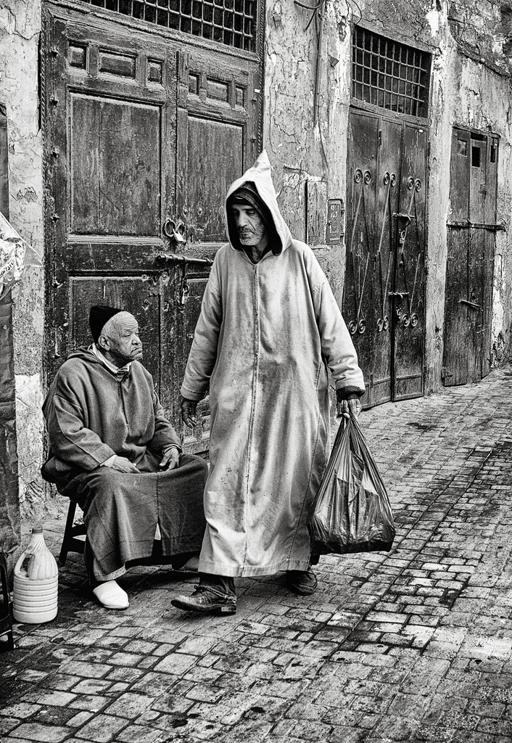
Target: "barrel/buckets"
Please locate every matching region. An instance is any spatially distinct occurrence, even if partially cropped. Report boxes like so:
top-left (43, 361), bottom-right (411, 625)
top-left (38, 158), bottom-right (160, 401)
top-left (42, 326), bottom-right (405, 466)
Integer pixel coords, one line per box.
top-left (13, 526), bottom-right (59, 624)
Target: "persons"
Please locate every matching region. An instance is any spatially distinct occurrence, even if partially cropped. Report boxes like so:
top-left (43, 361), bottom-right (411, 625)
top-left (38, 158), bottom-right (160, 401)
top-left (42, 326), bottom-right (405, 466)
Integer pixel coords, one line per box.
top-left (171, 148), bottom-right (367, 615)
top-left (41, 306), bottom-right (208, 609)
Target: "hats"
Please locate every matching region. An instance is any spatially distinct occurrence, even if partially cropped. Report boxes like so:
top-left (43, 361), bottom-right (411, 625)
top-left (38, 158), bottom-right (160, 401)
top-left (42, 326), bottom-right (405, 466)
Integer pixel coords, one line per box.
top-left (89, 304), bottom-right (123, 344)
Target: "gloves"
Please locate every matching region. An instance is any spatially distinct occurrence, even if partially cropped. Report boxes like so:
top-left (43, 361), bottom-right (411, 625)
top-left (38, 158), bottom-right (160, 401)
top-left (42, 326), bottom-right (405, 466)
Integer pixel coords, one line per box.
top-left (178, 397), bottom-right (198, 427)
top-left (113, 455), bottom-right (142, 473)
top-left (159, 448), bottom-right (179, 471)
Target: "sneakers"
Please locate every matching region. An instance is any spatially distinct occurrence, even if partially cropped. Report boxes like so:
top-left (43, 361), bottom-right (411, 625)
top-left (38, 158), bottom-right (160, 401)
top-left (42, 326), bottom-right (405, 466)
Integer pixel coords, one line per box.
top-left (91, 580), bottom-right (130, 610)
top-left (172, 587), bottom-right (236, 613)
top-left (286, 566), bottom-right (319, 592)
